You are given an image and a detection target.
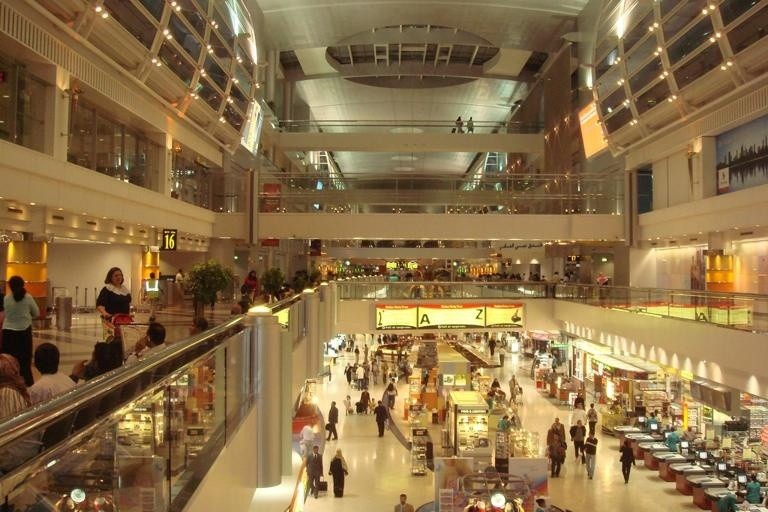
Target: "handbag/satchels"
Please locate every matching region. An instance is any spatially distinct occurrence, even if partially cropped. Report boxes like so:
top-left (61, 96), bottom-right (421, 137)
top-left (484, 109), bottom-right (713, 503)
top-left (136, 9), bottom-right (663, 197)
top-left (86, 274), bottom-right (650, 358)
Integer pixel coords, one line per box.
top-left (326, 424), bottom-right (329, 430)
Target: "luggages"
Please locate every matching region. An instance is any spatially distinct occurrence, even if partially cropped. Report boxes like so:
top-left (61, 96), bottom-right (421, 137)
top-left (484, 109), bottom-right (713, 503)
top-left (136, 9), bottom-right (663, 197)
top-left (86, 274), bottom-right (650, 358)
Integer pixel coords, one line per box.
top-left (319, 475), bottom-right (327, 491)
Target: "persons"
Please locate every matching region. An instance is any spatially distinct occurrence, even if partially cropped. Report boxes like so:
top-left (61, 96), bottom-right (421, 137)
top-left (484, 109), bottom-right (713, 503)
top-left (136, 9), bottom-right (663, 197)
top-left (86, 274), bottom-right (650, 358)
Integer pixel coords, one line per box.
top-left (1, 275), bottom-right (42, 387)
top-left (478, 271), bottom-right (608, 298)
top-left (0, 353), bottom-right (32, 425)
top-left (72, 342), bottom-right (118, 381)
top-left (545, 391), bottom-right (636, 484)
top-left (482, 332), bottom-right (508, 366)
top-left (361, 262), bottom-right (382, 276)
top-left (394, 494), bottom-right (414, 511)
top-left (498, 415), bottom-right (510, 431)
top-left (328, 448), bottom-right (350, 498)
top-left (26, 342), bottom-right (78, 404)
top-left (326, 379), bottom-right (399, 438)
top-left (121, 321), bottom-right (167, 367)
top-left (304, 446), bottom-right (323, 499)
top-left (466, 117), bottom-right (476, 134)
top-left (95, 265), bottom-right (134, 337)
top-left (149, 268), bottom-right (327, 328)
top-left (343, 334), bottom-right (414, 393)
top-left (490, 374), bottom-right (521, 408)
top-left (454, 116), bottom-right (463, 134)
